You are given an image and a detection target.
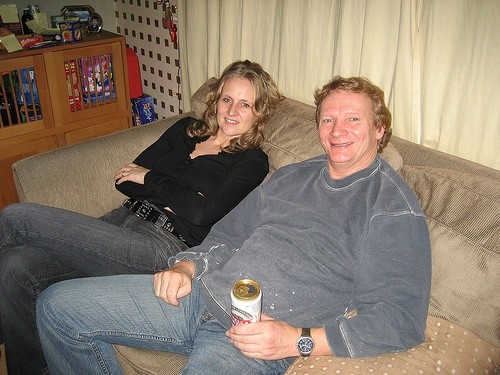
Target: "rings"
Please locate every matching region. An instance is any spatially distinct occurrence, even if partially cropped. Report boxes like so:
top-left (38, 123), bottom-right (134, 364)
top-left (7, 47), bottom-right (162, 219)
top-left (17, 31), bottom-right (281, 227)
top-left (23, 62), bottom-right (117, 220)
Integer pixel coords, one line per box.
top-left (122, 171), bottom-right (126, 177)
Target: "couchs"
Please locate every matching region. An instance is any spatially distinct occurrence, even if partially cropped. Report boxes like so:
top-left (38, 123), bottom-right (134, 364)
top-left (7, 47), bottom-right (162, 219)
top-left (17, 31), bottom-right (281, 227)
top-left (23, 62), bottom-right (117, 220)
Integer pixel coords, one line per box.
top-left (11, 77), bottom-right (500, 375)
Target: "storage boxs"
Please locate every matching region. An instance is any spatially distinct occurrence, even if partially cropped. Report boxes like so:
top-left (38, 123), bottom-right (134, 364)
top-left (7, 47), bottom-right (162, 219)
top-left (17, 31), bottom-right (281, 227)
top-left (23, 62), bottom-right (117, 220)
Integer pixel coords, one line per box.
top-left (132, 93), bottom-right (156, 125)
top-left (126, 48), bottom-right (143, 100)
top-left (59, 20), bottom-right (82, 44)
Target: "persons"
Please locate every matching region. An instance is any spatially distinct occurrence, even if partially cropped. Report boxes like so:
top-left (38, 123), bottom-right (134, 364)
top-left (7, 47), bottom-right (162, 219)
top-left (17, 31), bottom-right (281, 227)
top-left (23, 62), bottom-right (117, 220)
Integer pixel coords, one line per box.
top-left (35, 74), bottom-right (432, 375)
top-left (0, 58), bottom-right (286, 375)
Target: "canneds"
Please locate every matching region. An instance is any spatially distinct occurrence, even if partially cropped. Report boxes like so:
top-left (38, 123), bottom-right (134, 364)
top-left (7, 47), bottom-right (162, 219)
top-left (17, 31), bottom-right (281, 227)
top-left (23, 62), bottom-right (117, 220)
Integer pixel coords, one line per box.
top-left (28, 4), bottom-right (39, 17)
top-left (230, 278), bottom-right (263, 326)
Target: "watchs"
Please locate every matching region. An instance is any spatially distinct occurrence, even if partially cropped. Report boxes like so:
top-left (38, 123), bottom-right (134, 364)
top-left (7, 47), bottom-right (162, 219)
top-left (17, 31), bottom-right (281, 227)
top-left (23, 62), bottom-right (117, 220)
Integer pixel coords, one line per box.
top-left (296, 326), bottom-right (314, 358)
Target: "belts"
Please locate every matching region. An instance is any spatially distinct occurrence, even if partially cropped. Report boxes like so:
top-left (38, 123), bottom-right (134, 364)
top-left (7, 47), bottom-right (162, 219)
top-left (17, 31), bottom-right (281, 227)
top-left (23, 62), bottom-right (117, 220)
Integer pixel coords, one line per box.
top-left (124, 200), bottom-right (192, 249)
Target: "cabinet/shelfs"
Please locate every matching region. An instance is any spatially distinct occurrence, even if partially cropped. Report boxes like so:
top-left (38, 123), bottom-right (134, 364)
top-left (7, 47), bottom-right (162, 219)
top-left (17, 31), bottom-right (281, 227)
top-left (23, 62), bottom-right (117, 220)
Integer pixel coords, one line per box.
top-left (0, 29), bottom-right (134, 210)
